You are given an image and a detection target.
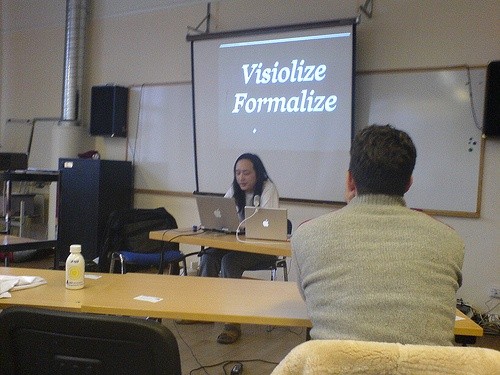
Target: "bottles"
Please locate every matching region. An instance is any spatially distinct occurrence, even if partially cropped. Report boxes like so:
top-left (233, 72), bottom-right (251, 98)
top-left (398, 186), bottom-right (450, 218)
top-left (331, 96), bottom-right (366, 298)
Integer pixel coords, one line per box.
top-left (65, 245), bottom-right (85, 290)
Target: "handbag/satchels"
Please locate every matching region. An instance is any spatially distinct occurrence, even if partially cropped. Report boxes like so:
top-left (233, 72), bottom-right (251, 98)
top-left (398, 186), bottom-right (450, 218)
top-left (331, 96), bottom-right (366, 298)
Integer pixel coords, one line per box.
top-left (107, 208), bottom-right (179, 253)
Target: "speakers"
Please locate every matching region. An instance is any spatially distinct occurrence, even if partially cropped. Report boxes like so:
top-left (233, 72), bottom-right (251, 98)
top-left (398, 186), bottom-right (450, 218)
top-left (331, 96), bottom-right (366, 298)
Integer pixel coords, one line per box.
top-left (89, 85), bottom-right (128, 136)
top-left (53, 158), bottom-right (134, 273)
top-left (482, 61), bottom-right (500, 137)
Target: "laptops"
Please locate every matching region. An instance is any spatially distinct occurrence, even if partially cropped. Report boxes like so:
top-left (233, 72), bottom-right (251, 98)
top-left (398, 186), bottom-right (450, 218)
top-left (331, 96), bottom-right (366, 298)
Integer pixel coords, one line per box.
top-left (244, 206), bottom-right (292, 240)
top-left (196, 196), bottom-right (244, 233)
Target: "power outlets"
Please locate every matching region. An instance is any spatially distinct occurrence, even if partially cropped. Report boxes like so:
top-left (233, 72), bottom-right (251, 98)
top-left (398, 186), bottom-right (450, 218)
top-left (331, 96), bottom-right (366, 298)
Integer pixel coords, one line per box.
top-left (489, 287), bottom-right (500, 298)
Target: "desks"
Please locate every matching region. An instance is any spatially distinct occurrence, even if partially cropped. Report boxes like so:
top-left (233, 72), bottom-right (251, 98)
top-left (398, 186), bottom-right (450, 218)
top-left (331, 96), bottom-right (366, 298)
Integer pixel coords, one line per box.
top-left (0, 170), bottom-right (59, 235)
top-left (150, 226), bottom-right (292, 274)
top-left (0, 235), bottom-right (57, 268)
top-left (0, 267), bottom-right (483, 346)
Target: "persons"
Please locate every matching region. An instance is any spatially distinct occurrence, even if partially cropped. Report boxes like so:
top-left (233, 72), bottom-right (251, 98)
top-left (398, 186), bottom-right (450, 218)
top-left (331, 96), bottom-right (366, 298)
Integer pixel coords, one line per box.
top-left (175, 152), bottom-right (279, 344)
top-left (290, 123), bottom-right (466, 345)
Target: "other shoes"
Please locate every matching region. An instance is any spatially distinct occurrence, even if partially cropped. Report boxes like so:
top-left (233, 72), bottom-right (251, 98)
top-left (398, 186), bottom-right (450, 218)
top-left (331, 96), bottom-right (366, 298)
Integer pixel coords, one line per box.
top-left (219, 324), bottom-right (242, 346)
top-left (175, 319), bottom-right (217, 326)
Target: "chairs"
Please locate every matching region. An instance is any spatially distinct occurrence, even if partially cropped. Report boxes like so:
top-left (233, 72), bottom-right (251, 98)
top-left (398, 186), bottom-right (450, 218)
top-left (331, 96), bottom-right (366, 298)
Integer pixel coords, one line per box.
top-left (271, 340), bottom-right (500, 375)
top-left (105, 206), bottom-right (188, 276)
top-left (243, 219), bottom-right (292, 280)
top-left (0, 307), bottom-right (181, 375)
top-left (0, 152), bottom-right (36, 226)
